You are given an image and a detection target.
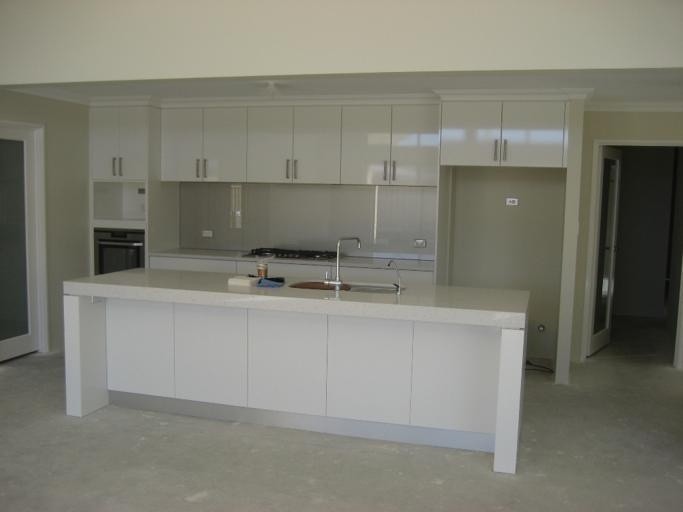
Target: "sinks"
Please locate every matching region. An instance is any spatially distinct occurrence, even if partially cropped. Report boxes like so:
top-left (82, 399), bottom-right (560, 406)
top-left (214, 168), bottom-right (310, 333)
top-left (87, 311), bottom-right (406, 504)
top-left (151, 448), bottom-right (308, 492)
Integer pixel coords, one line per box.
top-left (350, 285), bottom-right (398, 294)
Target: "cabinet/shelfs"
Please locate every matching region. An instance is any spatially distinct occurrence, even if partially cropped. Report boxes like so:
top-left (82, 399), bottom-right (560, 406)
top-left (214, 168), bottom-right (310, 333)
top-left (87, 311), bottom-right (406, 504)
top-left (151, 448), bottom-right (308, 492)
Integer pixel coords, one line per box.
top-left (161, 96), bottom-right (440, 188)
top-left (87, 101), bottom-right (181, 276)
top-left (441, 90), bottom-right (572, 169)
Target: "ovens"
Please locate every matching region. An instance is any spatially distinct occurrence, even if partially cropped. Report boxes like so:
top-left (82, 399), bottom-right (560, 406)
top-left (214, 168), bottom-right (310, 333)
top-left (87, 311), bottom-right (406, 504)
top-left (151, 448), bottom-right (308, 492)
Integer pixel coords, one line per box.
top-left (94, 228), bottom-right (144, 277)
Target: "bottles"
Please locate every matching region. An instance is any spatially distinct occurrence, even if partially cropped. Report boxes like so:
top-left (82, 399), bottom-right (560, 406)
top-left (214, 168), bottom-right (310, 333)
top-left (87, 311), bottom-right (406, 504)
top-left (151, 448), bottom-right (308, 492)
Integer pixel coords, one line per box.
top-left (257, 263), bottom-right (268, 278)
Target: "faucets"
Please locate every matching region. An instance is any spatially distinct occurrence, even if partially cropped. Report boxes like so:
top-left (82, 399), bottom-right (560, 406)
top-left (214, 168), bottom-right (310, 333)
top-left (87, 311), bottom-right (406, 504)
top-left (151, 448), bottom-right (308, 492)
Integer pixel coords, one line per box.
top-left (336, 237), bottom-right (361, 276)
top-left (389, 260), bottom-right (401, 293)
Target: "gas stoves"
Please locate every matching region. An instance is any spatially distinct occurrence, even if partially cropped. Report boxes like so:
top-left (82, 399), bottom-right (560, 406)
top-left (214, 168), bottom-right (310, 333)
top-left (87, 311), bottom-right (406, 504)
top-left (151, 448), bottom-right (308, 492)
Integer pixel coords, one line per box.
top-left (242, 247), bottom-right (341, 261)
